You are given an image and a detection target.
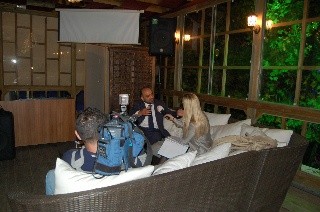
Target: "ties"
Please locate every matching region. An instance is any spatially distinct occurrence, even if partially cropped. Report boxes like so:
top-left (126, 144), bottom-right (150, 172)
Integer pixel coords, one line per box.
top-left (147, 104), bottom-right (155, 134)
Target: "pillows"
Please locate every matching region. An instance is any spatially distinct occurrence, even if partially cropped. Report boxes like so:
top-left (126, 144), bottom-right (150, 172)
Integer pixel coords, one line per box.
top-left (46, 111), bottom-right (294, 195)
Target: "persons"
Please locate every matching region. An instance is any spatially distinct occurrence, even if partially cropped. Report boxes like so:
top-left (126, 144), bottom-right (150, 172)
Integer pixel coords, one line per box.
top-left (62, 107), bottom-right (142, 171)
top-left (159, 93), bottom-right (213, 164)
top-left (129, 86), bottom-right (184, 144)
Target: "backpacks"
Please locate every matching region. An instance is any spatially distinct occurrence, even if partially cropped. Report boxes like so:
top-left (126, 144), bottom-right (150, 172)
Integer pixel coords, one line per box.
top-left (91, 114), bottom-right (153, 178)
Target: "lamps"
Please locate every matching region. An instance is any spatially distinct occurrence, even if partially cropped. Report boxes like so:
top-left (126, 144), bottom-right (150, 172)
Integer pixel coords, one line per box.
top-left (245, 12), bottom-right (260, 33)
top-left (263, 18), bottom-right (273, 38)
top-left (173, 31), bottom-right (180, 44)
top-left (183, 34), bottom-right (191, 46)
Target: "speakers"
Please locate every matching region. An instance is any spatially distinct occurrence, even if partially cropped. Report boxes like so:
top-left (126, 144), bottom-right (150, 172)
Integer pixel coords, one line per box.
top-left (148, 16), bottom-right (176, 57)
top-left (0, 110), bottom-right (16, 161)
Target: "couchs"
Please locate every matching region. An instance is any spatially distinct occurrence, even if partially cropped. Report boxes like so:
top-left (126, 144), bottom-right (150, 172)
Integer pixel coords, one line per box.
top-left (7, 124), bottom-right (309, 212)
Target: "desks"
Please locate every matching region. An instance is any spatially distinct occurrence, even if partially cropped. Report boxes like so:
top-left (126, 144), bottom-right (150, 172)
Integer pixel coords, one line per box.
top-left (0, 97), bottom-right (76, 147)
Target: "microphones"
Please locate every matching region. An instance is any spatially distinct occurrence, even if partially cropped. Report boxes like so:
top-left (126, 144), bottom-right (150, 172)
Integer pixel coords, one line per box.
top-left (157, 105), bottom-right (170, 120)
